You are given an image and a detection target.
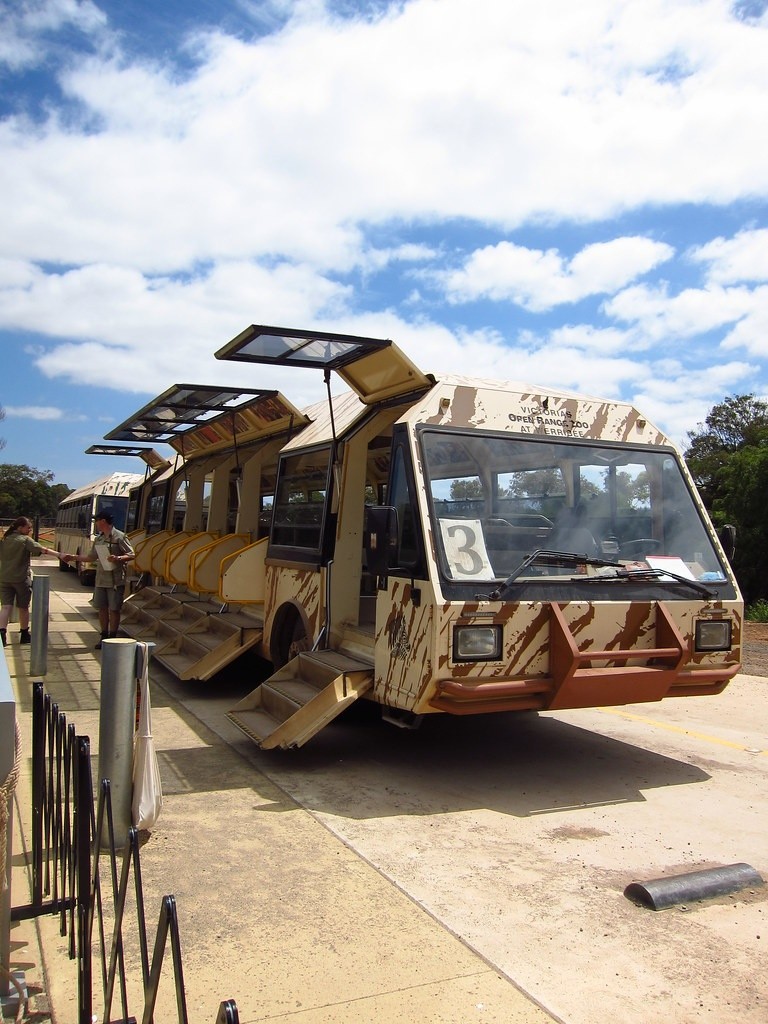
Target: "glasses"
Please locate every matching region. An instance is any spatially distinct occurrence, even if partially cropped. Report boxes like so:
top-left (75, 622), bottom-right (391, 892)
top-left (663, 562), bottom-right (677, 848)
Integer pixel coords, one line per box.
top-left (93, 518), bottom-right (101, 523)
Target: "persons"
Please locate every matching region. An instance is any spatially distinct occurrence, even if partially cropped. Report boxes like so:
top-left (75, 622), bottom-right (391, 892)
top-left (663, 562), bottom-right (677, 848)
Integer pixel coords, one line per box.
top-left (573, 505), bottom-right (602, 535)
top-left (66, 510), bottom-right (137, 649)
top-left (0, 516), bottom-right (72, 647)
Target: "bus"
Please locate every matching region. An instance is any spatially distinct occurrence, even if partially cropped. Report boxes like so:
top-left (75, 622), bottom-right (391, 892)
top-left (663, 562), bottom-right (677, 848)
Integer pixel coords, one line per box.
top-left (53, 471), bottom-right (144, 586)
top-left (85, 324), bottom-right (743, 751)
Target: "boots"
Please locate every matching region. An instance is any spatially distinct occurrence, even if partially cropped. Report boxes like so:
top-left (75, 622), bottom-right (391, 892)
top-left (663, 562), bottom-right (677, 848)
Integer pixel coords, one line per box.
top-left (20, 627), bottom-right (31, 644)
top-left (108, 632), bottom-right (116, 638)
top-left (0, 628), bottom-right (7, 647)
top-left (95, 631), bottom-right (107, 648)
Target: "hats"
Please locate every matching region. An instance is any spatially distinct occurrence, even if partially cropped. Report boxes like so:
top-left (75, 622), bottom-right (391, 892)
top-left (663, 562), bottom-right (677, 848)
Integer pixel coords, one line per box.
top-left (92, 511), bottom-right (113, 525)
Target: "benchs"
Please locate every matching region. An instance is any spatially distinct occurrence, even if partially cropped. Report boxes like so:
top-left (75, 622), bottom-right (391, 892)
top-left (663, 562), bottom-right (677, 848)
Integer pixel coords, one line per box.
top-left (169, 505), bottom-right (555, 565)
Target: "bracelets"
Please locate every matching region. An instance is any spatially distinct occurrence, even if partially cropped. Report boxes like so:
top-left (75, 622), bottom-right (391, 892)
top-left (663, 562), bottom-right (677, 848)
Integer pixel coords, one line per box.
top-left (118, 556), bottom-right (122, 561)
top-left (73, 554), bottom-right (76, 562)
top-left (58, 553), bottom-right (61, 559)
